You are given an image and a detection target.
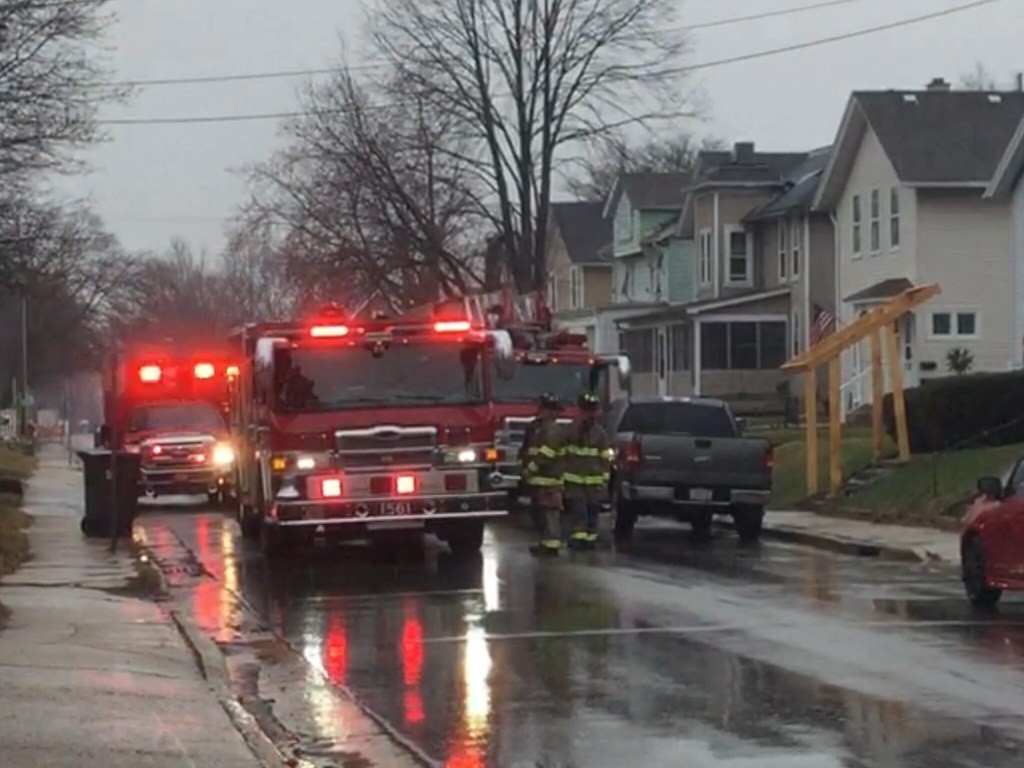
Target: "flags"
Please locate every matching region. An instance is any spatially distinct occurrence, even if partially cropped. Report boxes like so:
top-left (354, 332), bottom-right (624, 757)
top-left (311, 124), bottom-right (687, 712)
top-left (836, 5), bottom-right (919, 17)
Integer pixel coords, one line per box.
top-left (808, 304), bottom-right (837, 348)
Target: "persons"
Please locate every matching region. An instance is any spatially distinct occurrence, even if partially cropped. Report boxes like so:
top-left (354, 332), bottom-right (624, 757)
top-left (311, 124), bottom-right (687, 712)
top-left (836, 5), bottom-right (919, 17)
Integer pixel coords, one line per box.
top-left (515, 391), bottom-right (568, 557)
top-left (563, 393), bottom-right (618, 549)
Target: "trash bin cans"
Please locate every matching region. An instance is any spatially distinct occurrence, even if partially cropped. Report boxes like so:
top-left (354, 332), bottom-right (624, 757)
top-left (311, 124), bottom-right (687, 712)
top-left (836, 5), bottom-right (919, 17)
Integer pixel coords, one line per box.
top-left (74, 450), bottom-right (142, 538)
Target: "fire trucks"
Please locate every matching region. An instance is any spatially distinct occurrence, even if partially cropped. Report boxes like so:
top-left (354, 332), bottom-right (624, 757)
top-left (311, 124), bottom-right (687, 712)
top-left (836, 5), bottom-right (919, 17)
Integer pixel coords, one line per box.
top-left (489, 322), bottom-right (636, 515)
top-left (97, 339), bottom-right (242, 507)
top-left (228, 302), bottom-right (519, 555)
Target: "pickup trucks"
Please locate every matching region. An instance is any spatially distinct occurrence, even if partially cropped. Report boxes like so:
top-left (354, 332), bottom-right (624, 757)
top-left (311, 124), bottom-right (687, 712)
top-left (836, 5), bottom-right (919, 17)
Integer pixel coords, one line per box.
top-left (610, 395), bottom-right (777, 543)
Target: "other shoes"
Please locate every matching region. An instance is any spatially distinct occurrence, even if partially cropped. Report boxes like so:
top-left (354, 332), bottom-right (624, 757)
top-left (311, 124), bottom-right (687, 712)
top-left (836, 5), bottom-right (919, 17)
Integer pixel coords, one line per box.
top-left (588, 540), bottom-right (606, 549)
top-left (528, 544), bottom-right (558, 556)
top-left (569, 539), bottom-right (587, 551)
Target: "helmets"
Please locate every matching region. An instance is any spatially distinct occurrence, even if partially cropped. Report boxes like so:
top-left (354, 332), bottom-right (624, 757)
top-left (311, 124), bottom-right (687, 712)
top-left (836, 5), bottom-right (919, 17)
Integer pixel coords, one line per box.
top-left (539, 391), bottom-right (558, 406)
top-left (580, 393), bottom-right (600, 408)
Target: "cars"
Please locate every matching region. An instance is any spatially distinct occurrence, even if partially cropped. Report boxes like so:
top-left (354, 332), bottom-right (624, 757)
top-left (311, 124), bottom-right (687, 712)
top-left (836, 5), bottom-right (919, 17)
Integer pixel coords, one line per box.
top-left (959, 454), bottom-right (1024, 610)
